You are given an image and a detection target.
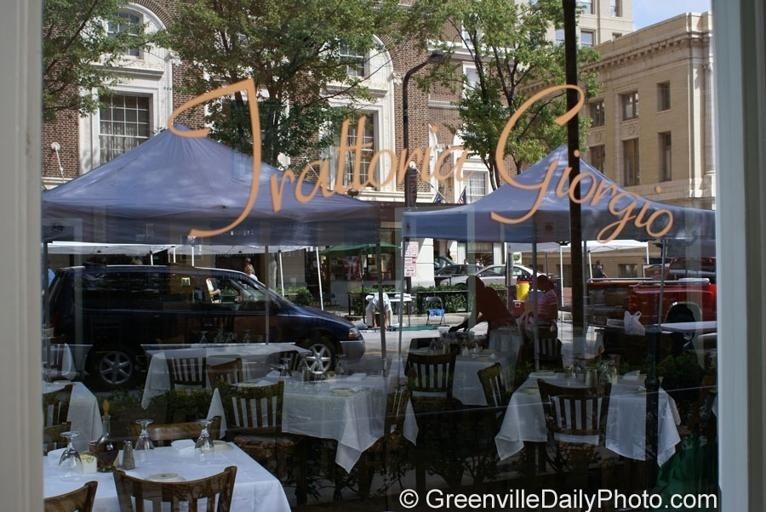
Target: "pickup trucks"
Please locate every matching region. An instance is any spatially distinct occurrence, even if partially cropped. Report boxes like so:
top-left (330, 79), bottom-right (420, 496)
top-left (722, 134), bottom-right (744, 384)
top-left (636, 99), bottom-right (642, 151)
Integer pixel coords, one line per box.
top-left (585, 255), bottom-right (719, 330)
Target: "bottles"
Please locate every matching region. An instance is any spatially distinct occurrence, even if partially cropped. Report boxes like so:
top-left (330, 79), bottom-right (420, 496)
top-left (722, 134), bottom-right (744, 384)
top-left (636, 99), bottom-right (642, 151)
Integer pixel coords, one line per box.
top-left (584, 368), bottom-right (599, 386)
top-left (94, 414), bottom-right (120, 475)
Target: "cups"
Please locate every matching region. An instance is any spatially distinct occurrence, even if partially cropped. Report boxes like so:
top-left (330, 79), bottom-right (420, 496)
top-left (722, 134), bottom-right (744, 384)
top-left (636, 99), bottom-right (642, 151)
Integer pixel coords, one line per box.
top-left (276, 353), bottom-right (349, 394)
top-left (427, 330), bottom-right (482, 358)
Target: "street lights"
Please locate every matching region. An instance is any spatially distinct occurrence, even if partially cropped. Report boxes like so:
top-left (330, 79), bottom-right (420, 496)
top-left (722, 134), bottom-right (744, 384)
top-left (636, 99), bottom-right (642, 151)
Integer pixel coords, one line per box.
top-left (400, 50), bottom-right (450, 202)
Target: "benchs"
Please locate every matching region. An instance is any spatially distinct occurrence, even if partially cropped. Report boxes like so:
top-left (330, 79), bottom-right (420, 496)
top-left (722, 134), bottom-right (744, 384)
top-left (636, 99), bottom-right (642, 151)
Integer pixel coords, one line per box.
top-left (416, 290), bottom-right (468, 314)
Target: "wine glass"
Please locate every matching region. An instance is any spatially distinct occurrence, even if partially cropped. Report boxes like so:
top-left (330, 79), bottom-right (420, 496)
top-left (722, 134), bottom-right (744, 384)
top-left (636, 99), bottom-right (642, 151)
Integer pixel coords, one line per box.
top-left (56, 430), bottom-right (84, 483)
top-left (134, 418), bottom-right (155, 468)
top-left (191, 417), bottom-right (216, 466)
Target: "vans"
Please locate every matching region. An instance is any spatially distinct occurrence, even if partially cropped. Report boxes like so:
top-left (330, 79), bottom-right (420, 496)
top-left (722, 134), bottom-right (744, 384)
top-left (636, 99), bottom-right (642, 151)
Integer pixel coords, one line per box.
top-left (41, 267), bottom-right (365, 389)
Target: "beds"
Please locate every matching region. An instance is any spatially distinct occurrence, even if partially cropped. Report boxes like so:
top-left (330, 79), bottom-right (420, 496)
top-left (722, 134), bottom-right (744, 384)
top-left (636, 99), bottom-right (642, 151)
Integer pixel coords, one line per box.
top-left (202, 358), bottom-right (418, 504)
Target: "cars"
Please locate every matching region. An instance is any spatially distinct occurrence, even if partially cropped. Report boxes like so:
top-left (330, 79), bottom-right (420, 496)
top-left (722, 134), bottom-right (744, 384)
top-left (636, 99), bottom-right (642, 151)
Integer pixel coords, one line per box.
top-left (441, 264), bottom-right (552, 293)
top-left (435, 263), bottom-right (484, 283)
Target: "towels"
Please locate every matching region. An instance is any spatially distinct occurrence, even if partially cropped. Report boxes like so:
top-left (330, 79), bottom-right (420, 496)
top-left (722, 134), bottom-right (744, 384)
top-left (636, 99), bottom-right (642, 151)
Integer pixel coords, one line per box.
top-left (266, 370), bottom-right (281, 378)
top-left (345, 371), bottom-right (367, 383)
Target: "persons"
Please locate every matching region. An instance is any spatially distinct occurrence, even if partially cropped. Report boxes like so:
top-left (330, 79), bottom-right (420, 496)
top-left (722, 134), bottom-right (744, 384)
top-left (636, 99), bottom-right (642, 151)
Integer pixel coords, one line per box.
top-left (537, 275), bottom-right (559, 366)
top-left (592, 265), bottom-right (608, 278)
top-left (476, 260), bottom-right (484, 271)
top-left (448, 276), bottom-right (522, 364)
top-left (334, 260), bottom-right (345, 280)
top-left (244, 257), bottom-right (254, 275)
top-left (204, 277), bottom-right (221, 303)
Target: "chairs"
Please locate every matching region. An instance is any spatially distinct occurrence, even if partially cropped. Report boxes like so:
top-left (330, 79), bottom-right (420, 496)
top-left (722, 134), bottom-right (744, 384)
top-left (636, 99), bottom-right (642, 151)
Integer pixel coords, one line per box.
top-left (41, 342), bottom-right (243, 512)
top-left (266, 350), bottom-right (300, 375)
top-left (306, 284), bottom-right (336, 307)
top-left (426, 296), bottom-right (446, 325)
top-left (334, 389), bottom-right (410, 512)
top-left (218, 382), bottom-right (307, 506)
